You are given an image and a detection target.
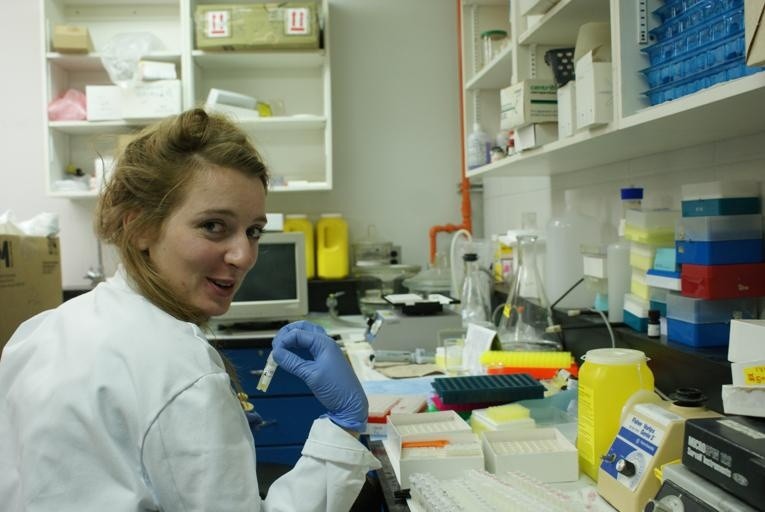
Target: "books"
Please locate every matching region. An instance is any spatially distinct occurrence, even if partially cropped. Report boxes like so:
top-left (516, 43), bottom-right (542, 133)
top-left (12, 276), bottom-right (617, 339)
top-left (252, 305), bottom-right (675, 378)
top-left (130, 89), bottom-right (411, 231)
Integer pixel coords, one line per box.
top-left (407, 467), bottom-right (600, 512)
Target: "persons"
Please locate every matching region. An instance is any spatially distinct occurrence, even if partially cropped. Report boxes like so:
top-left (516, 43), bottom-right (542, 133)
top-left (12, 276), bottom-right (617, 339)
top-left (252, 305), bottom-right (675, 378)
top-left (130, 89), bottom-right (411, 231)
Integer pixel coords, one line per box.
top-left (0, 108), bottom-right (383, 510)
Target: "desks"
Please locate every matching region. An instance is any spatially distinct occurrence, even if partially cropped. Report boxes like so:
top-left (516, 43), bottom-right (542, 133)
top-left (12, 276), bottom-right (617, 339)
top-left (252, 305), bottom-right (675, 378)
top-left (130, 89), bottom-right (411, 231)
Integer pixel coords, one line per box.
top-left (207, 311), bottom-right (616, 510)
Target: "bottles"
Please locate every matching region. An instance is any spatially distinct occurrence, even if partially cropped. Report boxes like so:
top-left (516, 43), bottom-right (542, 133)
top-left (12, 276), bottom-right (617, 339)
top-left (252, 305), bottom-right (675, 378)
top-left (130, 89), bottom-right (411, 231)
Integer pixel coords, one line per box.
top-left (575, 346), bottom-right (656, 485)
top-left (455, 252), bottom-right (493, 331)
top-left (282, 213), bottom-right (317, 281)
top-left (314, 211), bottom-right (351, 279)
top-left (495, 185), bottom-right (646, 354)
top-left (465, 122), bottom-right (492, 170)
top-left (480, 30), bottom-right (508, 70)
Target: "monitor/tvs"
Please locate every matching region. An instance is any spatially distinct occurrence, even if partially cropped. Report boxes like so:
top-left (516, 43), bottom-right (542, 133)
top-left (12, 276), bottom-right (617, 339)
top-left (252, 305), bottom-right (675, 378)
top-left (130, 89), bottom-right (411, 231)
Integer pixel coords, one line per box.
top-left (209, 231), bottom-right (309, 329)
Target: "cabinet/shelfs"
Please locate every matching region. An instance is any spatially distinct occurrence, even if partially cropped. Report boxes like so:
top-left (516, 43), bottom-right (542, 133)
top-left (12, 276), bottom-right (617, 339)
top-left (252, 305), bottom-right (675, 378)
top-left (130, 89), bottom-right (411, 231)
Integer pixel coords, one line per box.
top-left (38, 0), bottom-right (334, 198)
top-left (459, 0), bottom-right (763, 183)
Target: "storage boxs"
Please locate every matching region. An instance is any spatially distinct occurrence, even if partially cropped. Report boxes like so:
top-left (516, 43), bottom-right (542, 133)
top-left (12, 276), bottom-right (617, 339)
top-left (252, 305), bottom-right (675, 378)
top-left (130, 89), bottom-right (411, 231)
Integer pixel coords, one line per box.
top-left (81, 81), bottom-right (184, 120)
top-left (192, 4), bottom-right (320, 49)
top-left (495, 54), bottom-right (613, 153)
top-left (0, 233), bottom-right (62, 358)
